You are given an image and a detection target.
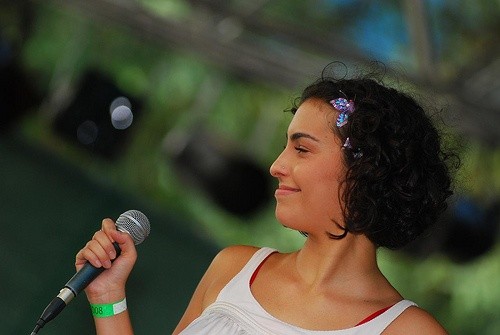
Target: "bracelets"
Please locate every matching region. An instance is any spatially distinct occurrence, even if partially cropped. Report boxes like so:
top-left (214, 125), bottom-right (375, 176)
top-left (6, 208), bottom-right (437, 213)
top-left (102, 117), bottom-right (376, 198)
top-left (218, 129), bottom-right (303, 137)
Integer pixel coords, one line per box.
top-left (90, 296), bottom-right (128, 317)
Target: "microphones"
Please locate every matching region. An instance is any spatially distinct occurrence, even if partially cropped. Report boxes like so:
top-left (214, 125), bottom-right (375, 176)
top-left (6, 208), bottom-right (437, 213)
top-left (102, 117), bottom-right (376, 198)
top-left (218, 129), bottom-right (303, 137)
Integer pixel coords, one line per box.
top-left (37, 209), bottom-right (149, 327)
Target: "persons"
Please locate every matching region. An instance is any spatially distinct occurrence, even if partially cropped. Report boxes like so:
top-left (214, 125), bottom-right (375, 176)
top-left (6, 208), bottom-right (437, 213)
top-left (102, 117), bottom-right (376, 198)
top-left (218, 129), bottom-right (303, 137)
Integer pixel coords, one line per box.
top-left (75, 62), bottom-right (462, 334)
